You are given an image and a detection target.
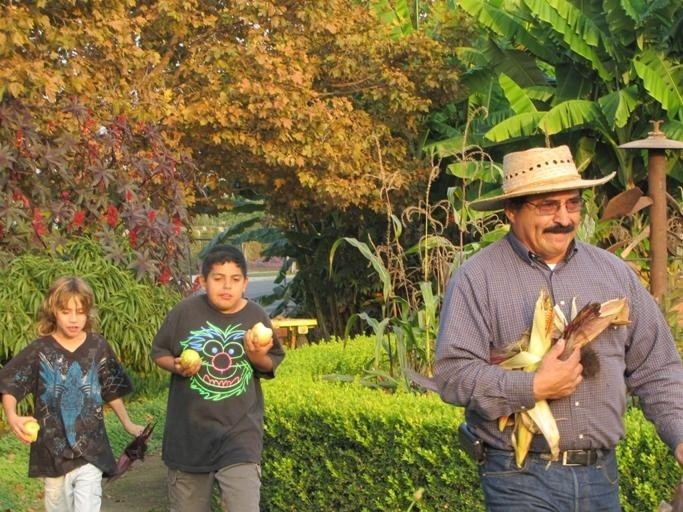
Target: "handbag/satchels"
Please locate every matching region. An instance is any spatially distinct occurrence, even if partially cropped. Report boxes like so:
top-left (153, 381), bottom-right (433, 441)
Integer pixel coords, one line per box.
top-left (458, 425), bottom-right (484, 461)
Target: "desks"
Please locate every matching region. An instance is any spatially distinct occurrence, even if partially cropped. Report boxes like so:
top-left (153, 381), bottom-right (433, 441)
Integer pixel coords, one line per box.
top-left (268, 318), bottom-right (318, 351)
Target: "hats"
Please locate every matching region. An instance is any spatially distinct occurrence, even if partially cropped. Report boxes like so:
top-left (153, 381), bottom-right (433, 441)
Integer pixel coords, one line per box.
top-left (468, 144), bottom-right (617, 212)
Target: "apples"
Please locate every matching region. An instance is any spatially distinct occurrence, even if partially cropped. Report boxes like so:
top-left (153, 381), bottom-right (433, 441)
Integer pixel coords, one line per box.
top-left (180, 350), bottom-right (200, 370)
top-left (253, 320), bottom-right (273, 348)
top-left (22, 421), bottom-right (39, 442)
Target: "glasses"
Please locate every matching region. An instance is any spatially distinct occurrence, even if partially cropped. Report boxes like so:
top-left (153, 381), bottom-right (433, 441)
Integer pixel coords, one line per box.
top-left (526, 197), bottom-right (584, 212)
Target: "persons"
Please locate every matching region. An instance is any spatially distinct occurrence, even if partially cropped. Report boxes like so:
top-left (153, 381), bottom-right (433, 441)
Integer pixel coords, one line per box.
top-left (150, 245), bottom-right (285, 512)
top-left (430, 145), bottom-right (683, 512)
top-left (1, 276), bottom-right (150, 512)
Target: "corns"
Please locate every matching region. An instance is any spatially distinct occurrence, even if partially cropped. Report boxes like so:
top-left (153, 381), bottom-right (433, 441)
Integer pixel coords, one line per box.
top-left (491, 285), bottom-right (632, 469)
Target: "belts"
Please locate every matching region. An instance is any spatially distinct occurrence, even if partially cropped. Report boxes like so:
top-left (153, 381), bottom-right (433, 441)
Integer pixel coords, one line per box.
top-left (528, 450), bottom-right (606, 466)
